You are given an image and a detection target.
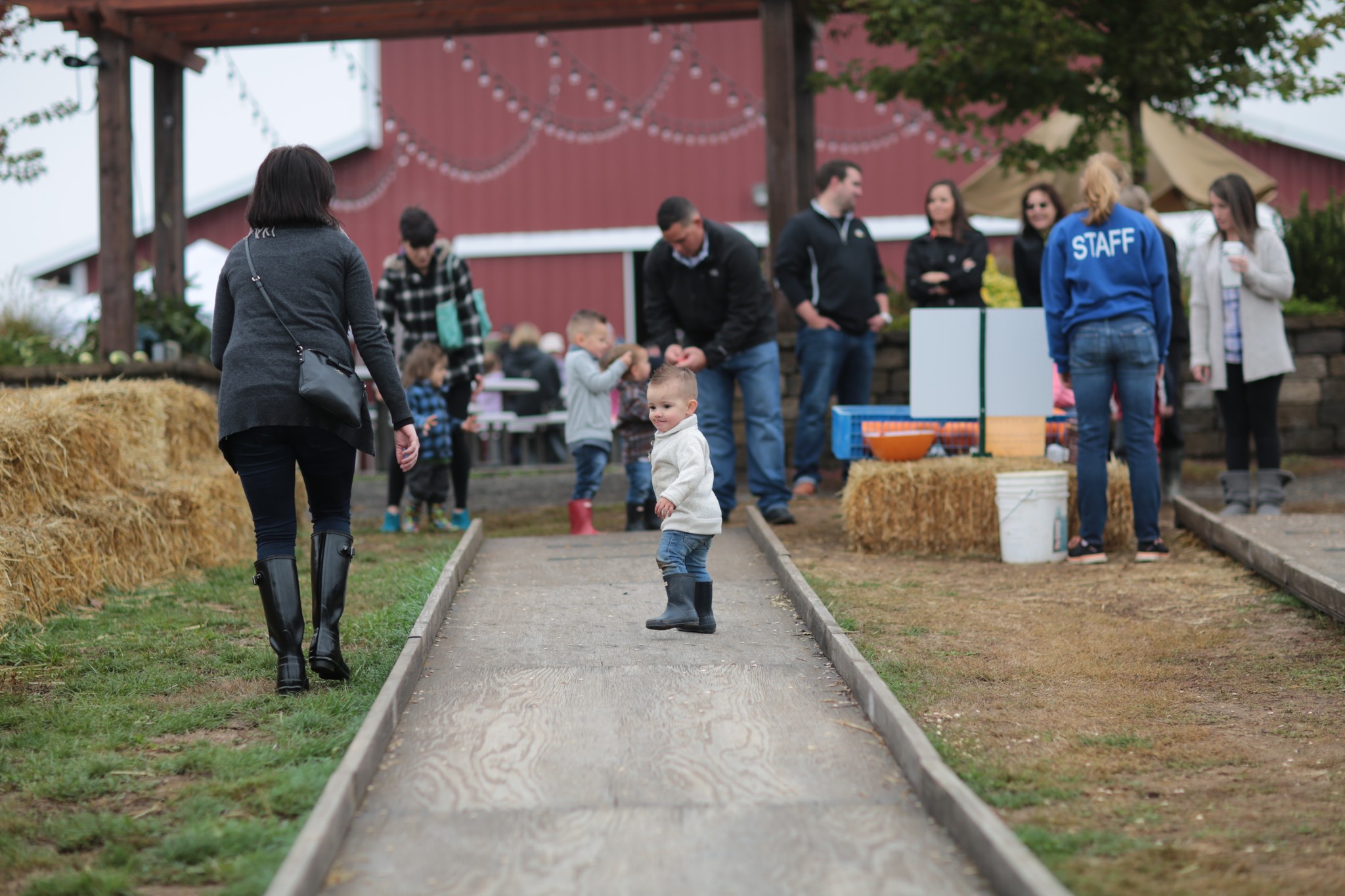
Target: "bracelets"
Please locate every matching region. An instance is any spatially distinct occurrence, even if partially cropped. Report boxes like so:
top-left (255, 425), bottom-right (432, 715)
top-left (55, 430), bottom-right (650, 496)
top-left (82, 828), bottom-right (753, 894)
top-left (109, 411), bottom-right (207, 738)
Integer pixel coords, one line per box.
top-left (878, 311), bottom-right (893, 326)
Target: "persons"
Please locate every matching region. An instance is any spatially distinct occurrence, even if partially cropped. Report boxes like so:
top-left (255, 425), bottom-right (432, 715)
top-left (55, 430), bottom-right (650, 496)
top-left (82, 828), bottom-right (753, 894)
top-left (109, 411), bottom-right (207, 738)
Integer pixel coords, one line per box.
top-left (646, 361), bottom-right (722, 635)
top-left (1010, 179), bottom-right (1068, 307)
top-left (599, 342), bottom-right (687, 533)
top-left (399, 340), bottom-right (485, 534)
top-left (211, 143), bottom-right (419, 697)
top-left (903, 177), bottom-right (988, 308)
top-left (770, 159), bottom-right (893, 496)
top-left (1040, 150), bottom-right (1174, 566)
top-left (643, 195), bottom-right (797, 524)
top-left (375, 206), bottom-right (487, 534)
top-left (469, 313), bottom-right (625, 466)
top-left (1119, 184), bottom-right (1193, 502)
top-left (1188, 172), bottom-right (1299, 523)
top-left (562, 308), bottom-right (632, 535)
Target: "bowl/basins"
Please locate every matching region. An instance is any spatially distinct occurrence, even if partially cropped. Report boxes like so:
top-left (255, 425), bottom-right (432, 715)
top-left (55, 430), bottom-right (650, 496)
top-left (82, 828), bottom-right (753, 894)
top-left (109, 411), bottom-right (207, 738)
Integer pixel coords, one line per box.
top-left (938, 433), bottom-right (977, 456)
top-left (864, 430), bottom-right (935, 462)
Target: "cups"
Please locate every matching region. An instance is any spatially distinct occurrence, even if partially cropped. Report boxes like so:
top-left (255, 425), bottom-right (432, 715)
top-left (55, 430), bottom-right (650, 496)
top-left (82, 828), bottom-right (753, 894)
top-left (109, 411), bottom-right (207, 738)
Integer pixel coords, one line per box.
top-left (1222, 240), bottom-right (1243, 288)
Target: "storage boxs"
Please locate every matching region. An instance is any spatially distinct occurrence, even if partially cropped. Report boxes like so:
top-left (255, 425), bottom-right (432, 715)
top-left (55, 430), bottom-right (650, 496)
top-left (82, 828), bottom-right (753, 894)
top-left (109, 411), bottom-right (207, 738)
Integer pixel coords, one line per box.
top-left (831, 405), bottom-right (978, 462)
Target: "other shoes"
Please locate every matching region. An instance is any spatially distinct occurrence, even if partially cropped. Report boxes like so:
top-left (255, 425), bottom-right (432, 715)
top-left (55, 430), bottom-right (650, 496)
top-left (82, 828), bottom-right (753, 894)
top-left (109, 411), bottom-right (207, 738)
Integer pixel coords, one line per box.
top-left (764, 501), bottom-right (793, 522)
top-left (1137, 536), bottom-right (1172, 564)
top-left (451, 505), bottom-right (471, 531)
top-left (428, 502), bottom-right (459, 533)
top-left (383, 509), bottom-right (400, 532)
top-left (1067, 540), bottom-right (1109, 564)
top-left (402, 501), bottom-right (423, 534)
top-left (794, 482), bottom-right (815, 495)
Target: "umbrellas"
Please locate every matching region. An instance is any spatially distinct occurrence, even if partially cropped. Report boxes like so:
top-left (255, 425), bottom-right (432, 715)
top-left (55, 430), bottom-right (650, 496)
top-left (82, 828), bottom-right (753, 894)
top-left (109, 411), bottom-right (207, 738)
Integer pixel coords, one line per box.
top-left (955, 77), bottom-right (1280, 209)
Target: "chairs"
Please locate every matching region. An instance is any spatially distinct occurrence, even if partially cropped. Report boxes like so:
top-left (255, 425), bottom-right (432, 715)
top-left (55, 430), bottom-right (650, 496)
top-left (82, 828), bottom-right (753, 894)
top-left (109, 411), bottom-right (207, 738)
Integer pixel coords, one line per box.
top-left (467, 377), bottom-right (566, 464)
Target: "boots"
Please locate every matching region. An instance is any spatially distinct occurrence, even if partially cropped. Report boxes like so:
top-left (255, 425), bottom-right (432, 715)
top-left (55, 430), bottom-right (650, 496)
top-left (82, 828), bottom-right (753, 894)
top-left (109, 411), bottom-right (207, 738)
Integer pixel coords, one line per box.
top-left (645, 573), bottom-right (718, 632)
top-left (1218, 468), bottom-right (1289, 515)
top-left (567, 499), bottom-right (600, 534)
top-left (625, 501), bottom-right (662, 530)
top-left (253, 554), bottom-right (308, 690)
top-left (310, 531), bottom-right (355, 681)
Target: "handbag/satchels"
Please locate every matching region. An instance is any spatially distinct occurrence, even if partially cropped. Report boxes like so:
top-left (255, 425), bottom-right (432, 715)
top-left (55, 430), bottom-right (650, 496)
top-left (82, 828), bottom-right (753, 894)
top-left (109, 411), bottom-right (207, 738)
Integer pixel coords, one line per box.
top-left (436, 249), bottom-right (491, 352)
top-left (295, 345), bottom-right (367, 429)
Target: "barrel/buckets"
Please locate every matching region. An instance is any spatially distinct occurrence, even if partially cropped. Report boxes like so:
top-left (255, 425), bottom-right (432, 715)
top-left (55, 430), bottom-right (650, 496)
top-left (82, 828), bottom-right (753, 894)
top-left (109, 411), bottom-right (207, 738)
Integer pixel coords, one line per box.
top-left (994, 470), bottom-right (1069, 564)
